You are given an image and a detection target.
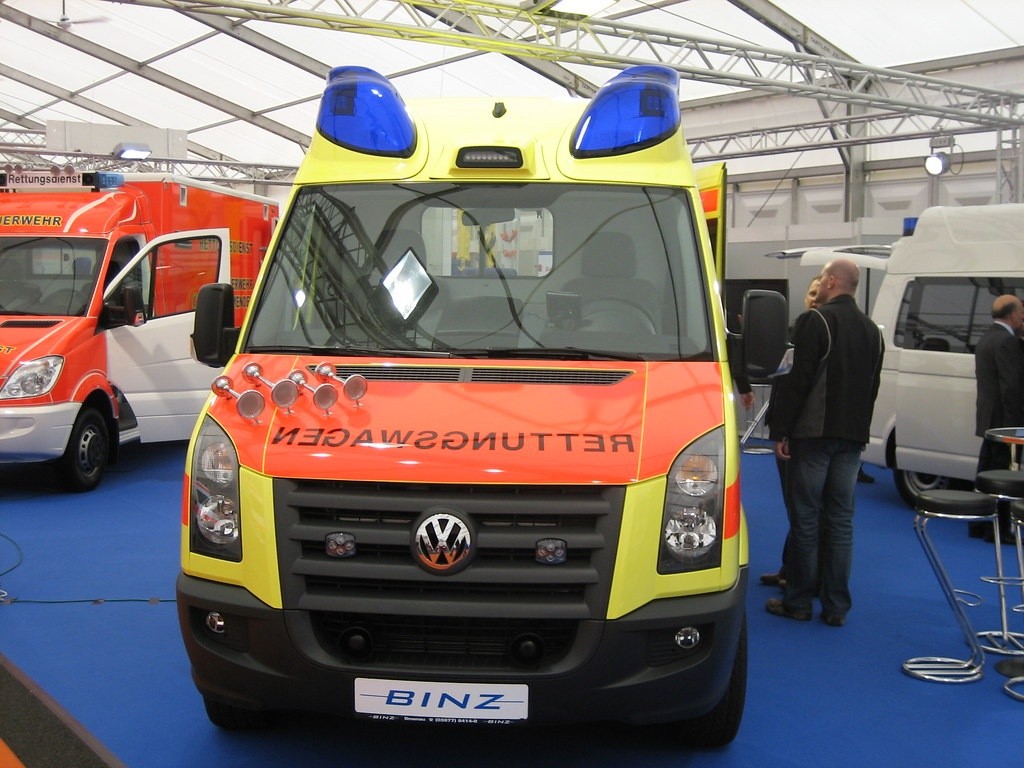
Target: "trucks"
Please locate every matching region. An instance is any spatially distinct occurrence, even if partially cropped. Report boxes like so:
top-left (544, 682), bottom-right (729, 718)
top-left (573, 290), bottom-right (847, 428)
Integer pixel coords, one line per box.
top-left (0, 163), bottom-right (281, 495)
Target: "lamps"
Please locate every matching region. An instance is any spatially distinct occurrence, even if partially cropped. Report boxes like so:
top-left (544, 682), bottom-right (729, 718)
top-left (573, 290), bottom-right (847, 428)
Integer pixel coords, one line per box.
top-left (109, 142), bottom-right (152, 160)
top-left (4, 162), bottom-right (79, 175)
top-left (923, 124), bottom-right (965, 176)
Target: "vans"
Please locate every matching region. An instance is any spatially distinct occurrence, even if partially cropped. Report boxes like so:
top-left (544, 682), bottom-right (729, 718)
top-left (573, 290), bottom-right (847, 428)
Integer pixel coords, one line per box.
top-left (763, 202), bottom-right (1024, 510)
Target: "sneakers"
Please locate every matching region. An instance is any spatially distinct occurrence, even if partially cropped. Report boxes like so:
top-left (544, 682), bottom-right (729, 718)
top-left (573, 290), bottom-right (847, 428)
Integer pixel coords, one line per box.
top-left (759, 572), bottom-right (786, 592)
top-left (766, 598), bottom-right (813, 620)
top-left (820, 608), bottom-right (844, 626)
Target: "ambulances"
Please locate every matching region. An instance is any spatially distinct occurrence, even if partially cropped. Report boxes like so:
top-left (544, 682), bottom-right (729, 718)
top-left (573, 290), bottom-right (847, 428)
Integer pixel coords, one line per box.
top-left (177, 66), bottom-right (791, 758)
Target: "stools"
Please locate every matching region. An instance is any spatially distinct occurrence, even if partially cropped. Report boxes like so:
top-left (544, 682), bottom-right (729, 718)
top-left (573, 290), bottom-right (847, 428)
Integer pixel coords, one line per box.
top-left (901, 470), bottom-right (1024, 701)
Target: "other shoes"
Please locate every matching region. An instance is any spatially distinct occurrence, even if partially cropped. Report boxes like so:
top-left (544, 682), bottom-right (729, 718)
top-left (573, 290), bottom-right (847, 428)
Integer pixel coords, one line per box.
top-left (968, 528), bottom-right (1016, 544)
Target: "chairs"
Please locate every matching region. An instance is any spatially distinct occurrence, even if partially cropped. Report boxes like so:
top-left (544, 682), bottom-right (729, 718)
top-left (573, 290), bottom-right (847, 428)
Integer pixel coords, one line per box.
top-left (361, 230), bottom-right (450, 319)
top-left (562, 232), bottom-right (664, 327)
top-left (83, 261), bottom-right (124, 305)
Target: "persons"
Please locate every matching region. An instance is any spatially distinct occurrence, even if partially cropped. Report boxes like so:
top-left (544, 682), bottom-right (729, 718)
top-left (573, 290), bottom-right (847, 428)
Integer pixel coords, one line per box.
top-left (727, 312), bottom-right (754, 409)
top-left (970, 294), bottom-right (1024, 542)
top-left (759, 258), bottom-right (885, 624)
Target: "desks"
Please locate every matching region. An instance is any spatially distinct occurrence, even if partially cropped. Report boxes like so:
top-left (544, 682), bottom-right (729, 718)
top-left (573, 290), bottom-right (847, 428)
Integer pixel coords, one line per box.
top-left (985, 427), bottom-right (1024, 678)
